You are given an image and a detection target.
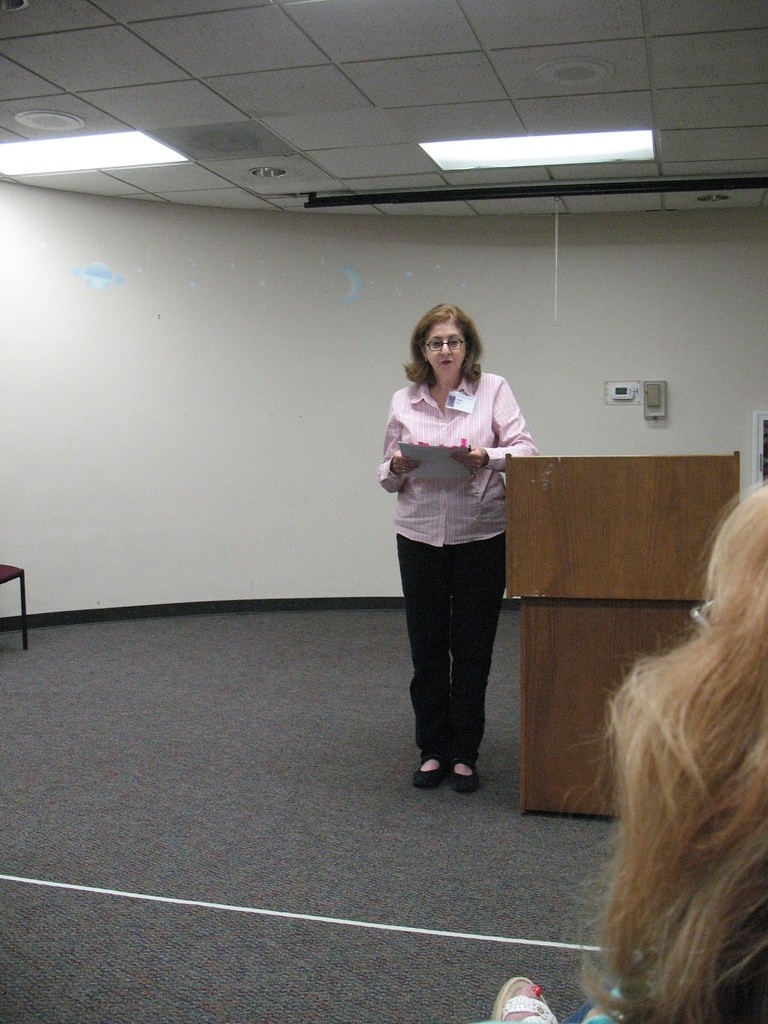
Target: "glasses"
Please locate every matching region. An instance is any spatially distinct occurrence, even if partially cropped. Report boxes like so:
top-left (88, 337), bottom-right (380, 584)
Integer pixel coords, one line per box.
top-left (425, 338), bottom-right (467, 351)
top-left (690, 601), bottom-right (717, 632)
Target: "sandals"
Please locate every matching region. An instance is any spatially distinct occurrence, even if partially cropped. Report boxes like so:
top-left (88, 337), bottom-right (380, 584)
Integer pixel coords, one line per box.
top-left (491, 977), bottom-right (557, 1024)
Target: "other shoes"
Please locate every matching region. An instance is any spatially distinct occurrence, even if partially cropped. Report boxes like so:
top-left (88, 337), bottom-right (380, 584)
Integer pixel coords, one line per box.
top-left (413, 757), bottom-right (446, 788)
top-left (449, 758), bottom-right (478, 791)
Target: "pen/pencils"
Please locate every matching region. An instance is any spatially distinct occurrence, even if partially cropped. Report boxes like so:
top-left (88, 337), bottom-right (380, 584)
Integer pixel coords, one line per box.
top-left (468, 444), bottom-right (475, 474)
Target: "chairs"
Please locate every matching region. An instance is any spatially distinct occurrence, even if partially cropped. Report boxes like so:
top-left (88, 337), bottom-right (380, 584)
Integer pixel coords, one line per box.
top-left (0, 564), bottom-right (28, 650)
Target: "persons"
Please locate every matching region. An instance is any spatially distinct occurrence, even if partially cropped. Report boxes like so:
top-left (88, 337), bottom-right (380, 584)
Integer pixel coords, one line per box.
top-left (378, 302), bottom-right (538, 795)
top-left (492, 478), bottom-right (767, 1024)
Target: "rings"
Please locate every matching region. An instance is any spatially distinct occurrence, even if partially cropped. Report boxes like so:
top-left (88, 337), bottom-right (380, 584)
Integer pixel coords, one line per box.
top-left (403, 466), bottom-right (406, 470)
top-left (402, 469), bottom-right (406, 472)
